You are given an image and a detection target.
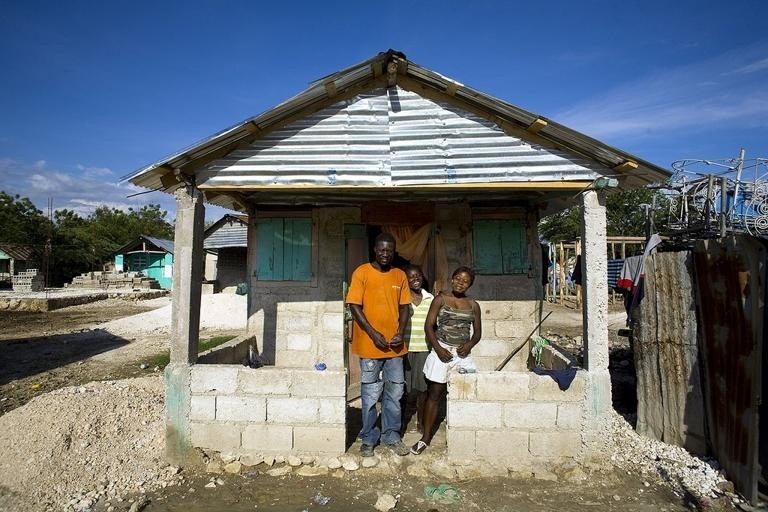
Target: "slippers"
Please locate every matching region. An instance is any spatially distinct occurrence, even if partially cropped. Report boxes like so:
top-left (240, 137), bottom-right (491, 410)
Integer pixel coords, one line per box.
top-left (410, 440), bottom-right (428, 455)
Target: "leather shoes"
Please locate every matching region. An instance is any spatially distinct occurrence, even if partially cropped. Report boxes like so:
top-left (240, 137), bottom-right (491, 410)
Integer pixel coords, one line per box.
top-left (360, 442), bottom-right (375, 456)
top-left (386, 439), bottom-right (410, 455)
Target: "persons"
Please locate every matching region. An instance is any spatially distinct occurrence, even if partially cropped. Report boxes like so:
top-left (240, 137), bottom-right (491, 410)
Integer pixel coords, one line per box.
top-left (410, 266), bottom-right (482, 456)
top-left (345, 232), bottom-right (414, 457)
top-left (404, 266), bottom-right (434, 434)
